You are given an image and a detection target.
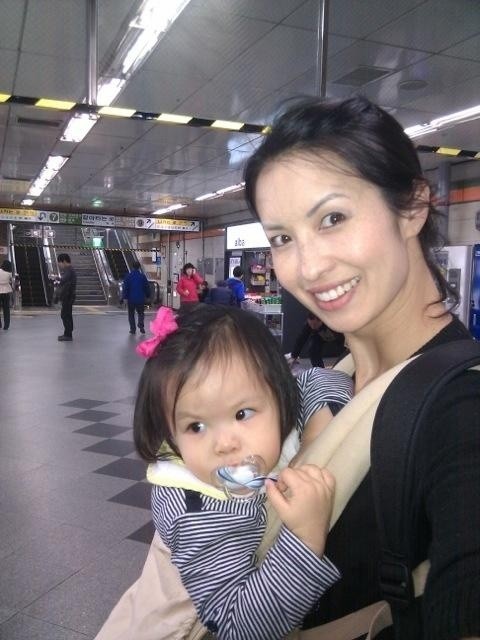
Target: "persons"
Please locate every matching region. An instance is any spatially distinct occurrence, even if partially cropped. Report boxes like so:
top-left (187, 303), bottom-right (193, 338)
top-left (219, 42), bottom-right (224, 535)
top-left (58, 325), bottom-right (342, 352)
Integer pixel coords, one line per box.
top-left (121, 261), bottom-right (151, 334)
top-left (242, 95), bottom-right (480, 640)
top-left (55, 253), bottom-right (77, 341)
top-left (0, 260), bottom-right (13, 330)
top-left (176, 262), bottom-right (254, 308)
top-left (288, 311), bottom-right (347, 368)
top-left (92, 305), bottom-right (356, 640)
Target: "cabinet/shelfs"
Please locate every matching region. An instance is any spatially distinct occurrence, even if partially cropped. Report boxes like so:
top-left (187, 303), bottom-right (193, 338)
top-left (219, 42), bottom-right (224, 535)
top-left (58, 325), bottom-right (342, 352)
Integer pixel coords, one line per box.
top-left (241, 300), bottom-right (284, 345)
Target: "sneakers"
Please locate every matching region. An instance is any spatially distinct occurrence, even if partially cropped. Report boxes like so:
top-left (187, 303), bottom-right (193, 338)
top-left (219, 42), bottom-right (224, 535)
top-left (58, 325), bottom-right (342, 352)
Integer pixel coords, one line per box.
top-left (57, 334), bottom-right (73, 341)
top-left (139, 327), bottom-right (145, 334)
top-left (129, 329), bottom-right (136, 335)
